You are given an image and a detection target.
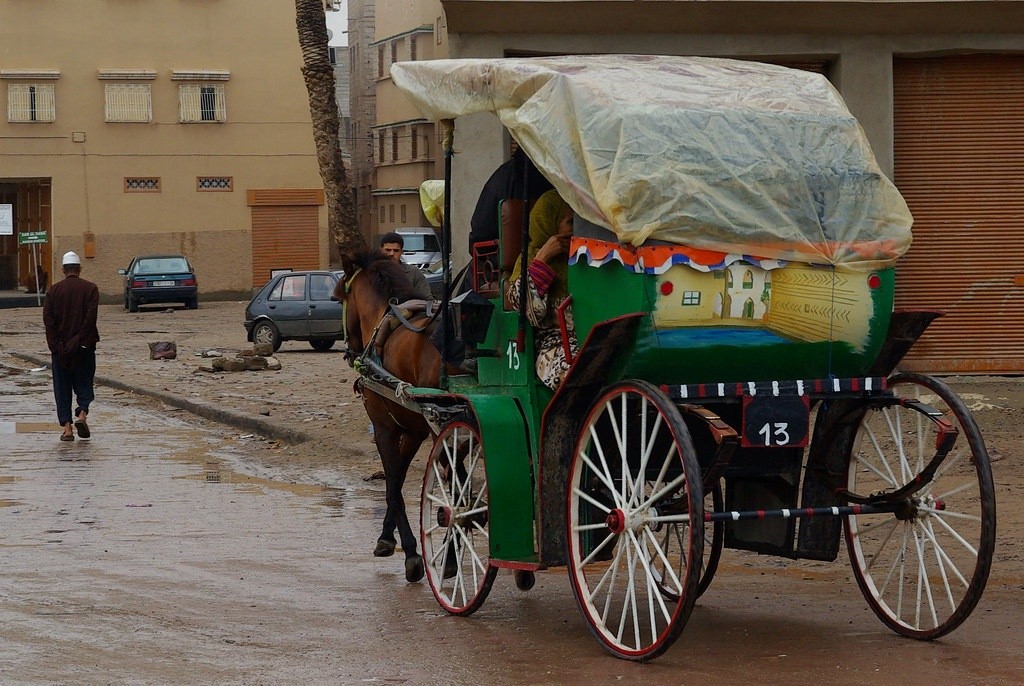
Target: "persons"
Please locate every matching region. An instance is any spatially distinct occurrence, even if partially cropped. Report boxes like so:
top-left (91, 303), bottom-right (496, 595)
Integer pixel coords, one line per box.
top-left (372, 233), bottom-right (435, 443)
top-left (43, 251), bottom-right (100, 439)
top-left (507, 189), bottom-right (581, 392)
top-left (461, 145), bottom-right (557, 373)
top-left (27, 265), bottom-right (43, 293)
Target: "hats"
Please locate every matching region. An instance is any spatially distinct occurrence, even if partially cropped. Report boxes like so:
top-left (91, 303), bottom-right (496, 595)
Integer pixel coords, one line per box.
top-left (62, 250), bottom-right (81, 265)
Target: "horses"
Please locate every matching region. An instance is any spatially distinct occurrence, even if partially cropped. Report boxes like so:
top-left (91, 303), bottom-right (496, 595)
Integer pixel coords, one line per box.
top-left (330, 233), bottom-right (536, 593)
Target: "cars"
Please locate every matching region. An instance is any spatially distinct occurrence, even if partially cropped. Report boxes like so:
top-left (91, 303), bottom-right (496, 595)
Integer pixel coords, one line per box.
top-left (394, 227), bottom-right (442, 269)
top-left (117, 255), bottom-right (198, 313)
top-left (244, 269), bottom-right (345, 352)
top-left (422, 253), bottom-right (452, 296)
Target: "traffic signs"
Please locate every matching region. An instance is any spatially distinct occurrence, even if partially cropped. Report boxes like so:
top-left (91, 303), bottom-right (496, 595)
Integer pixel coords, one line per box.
top-left (19, 230), bottom-right (47, 245)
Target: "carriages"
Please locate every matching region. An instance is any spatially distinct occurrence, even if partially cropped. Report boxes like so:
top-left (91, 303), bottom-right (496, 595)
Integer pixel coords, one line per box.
top-left (333, 53), bottom-right (997, 662)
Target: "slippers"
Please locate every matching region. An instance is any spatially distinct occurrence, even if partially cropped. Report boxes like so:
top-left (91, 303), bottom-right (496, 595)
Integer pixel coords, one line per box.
top-left (60, 432), bottom-right (74, 441)
top-left (74, 420), bottom-right (91, 438)
